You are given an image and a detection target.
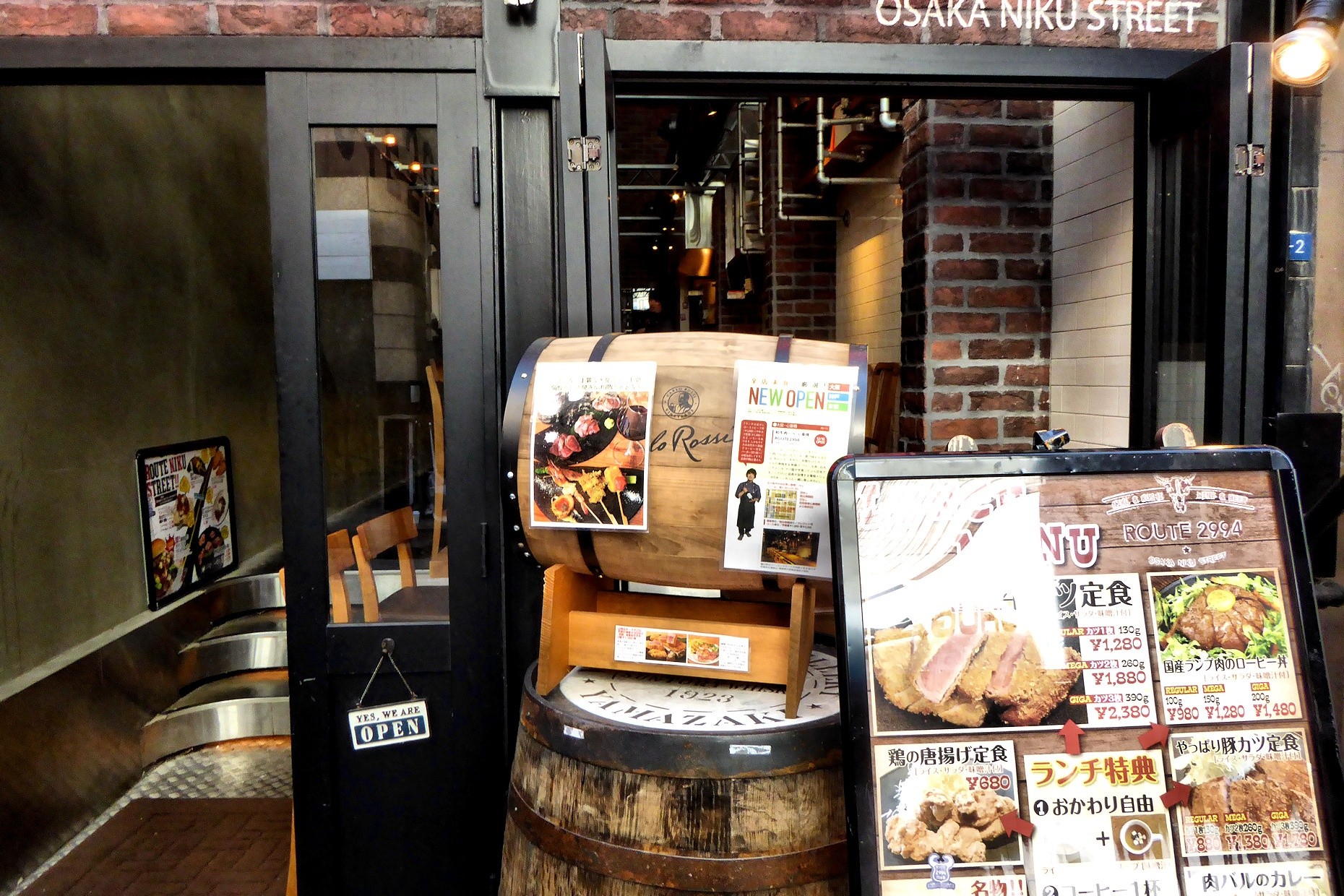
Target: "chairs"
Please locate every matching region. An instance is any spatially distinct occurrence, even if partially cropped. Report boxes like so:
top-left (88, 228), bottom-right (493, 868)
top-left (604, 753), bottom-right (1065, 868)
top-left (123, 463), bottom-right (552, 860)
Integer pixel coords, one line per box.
top-left (276, 506), bottom-right (453, 622)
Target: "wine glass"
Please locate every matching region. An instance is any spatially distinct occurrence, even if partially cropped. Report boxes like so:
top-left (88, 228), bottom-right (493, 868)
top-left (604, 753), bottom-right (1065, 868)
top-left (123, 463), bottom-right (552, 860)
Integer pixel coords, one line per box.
top-left (613, 391), bottom-right (649, 468)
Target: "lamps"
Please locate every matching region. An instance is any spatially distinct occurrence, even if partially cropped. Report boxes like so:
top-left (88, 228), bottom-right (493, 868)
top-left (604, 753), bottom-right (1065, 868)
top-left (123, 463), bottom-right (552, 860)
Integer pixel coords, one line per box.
top-left (394, 161), bottom-right (422, 172)
top-left (365, 132), bottom-right (397, 146)
top-left (1273, 0), bottom-right (1344, 87)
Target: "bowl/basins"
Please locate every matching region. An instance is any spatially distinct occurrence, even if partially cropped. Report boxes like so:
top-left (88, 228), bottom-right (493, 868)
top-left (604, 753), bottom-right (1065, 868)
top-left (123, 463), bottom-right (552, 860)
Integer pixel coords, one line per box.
top-left (1119, 819), bottom-right (1153, 855)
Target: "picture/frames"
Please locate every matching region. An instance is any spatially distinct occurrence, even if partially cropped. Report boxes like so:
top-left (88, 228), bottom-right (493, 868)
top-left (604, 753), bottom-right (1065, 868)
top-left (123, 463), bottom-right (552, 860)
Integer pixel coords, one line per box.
top-left (136, 436), bottom-right (238, 611)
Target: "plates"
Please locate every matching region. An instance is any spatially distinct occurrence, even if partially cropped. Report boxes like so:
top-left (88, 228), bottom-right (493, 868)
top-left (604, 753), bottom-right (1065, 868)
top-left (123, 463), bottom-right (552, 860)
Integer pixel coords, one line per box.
top-left (687, 642), bottom-right (719, 665)
top-left (1154, 573), bottom-right (1276, 601)
top-left (211, 490), bottom-right (229, 526)
top-left (532, 462), bottom-right (644, 524)
top-left (645, 635), bottom-right (686, 663)
top-left (194, 527), bottom-right (224, 581)
top-left (534, 392), bottom-right (622, 466)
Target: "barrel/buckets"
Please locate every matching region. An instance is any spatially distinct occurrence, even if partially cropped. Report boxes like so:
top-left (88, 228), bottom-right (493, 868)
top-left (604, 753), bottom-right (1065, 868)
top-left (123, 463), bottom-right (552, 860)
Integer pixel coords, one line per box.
top-left (497, 633), bottom-right (850, 896)
top-left (498, 330), bottom-right (868, 591)
